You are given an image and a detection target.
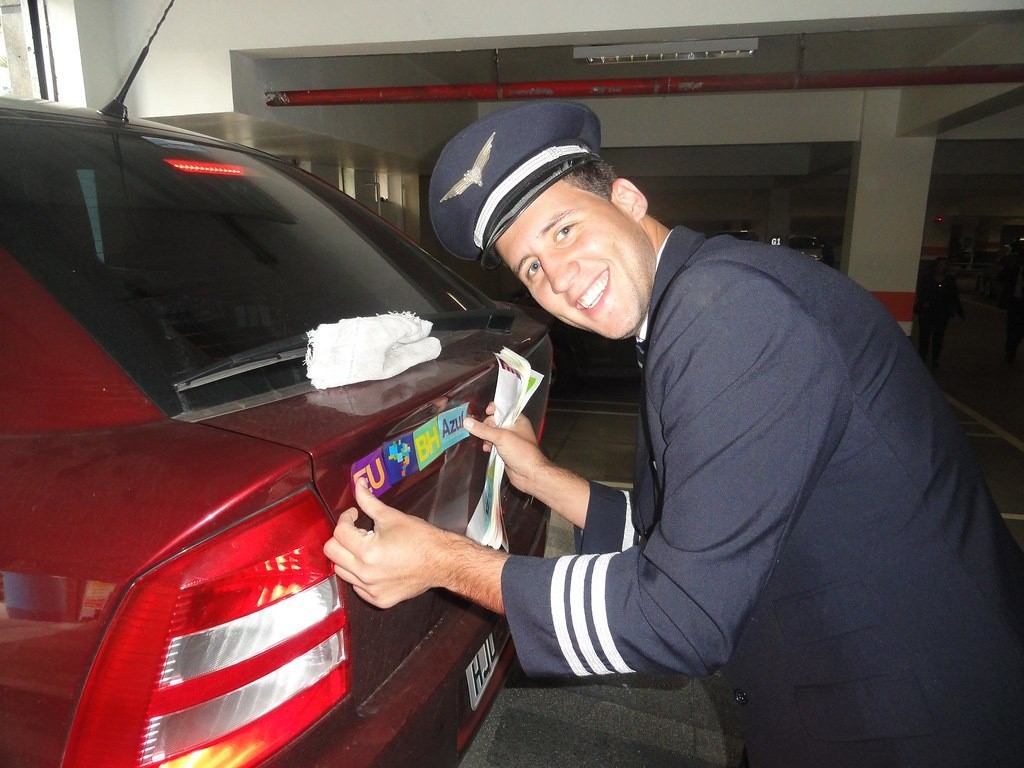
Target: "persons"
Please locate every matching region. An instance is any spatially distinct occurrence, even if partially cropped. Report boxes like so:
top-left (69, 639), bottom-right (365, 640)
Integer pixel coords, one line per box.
top-left (912, 256), bottom-right (965, 370)
top-left (322, 99), bottom-right (1024, 768)
top-left (950, 232), bottom-right (973, 262)
top-left (996, 249), bottom-right (1024, 364)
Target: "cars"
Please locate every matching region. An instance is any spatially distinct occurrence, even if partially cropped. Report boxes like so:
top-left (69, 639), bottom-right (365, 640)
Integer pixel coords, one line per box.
top-left (508, 286), bottom-right (540, 308)
top-left (713, 230), bottom-right (824, 264)
top-left (1, 98), bottom-right (551, 768)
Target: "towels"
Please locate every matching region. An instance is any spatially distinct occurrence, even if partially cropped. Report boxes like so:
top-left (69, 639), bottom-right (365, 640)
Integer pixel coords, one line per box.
top-left (302, 310), bottom-right (441, 390)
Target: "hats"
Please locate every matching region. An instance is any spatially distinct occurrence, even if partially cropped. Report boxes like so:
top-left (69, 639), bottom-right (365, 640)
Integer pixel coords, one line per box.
top-left (428, 97), bottom-right (601, 270)
